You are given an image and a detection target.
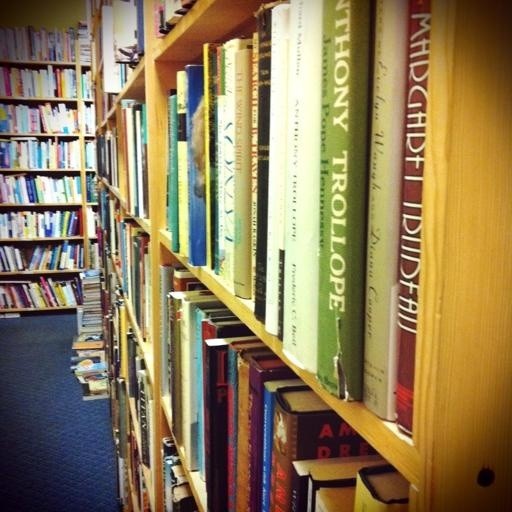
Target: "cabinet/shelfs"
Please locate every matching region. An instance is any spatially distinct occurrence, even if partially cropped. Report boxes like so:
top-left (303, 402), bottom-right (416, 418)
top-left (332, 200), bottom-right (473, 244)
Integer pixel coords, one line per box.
top-left (94, 0), bottom-right (511, 512)
top-left (0, 41), bottom-right (96, 313)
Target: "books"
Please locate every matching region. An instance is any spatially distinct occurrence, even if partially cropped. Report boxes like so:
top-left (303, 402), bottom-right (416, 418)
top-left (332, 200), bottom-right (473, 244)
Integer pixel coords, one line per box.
top-left (68, 267), bottom-right (110, 403)
top-left (0, 18), bottom-right (109, 312)
top-left (91, 1), bottom-right (434, 511)
